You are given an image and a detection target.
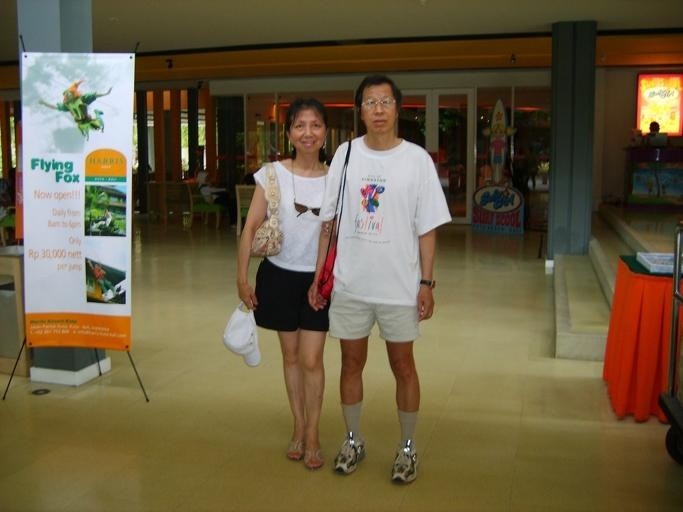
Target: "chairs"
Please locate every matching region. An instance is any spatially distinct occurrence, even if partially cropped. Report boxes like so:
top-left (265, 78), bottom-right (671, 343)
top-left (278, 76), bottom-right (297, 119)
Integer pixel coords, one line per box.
top-left (187, 183), bottom-right (255, 237)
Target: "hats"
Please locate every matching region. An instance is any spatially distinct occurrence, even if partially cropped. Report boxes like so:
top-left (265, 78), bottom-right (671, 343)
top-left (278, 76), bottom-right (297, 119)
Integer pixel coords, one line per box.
top-left (222, 299), bottom-right (262, 367)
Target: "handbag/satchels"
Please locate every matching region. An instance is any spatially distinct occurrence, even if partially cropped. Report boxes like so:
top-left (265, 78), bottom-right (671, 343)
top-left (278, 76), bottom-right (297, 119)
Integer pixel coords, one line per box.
top-left (249, 216), bottom-right (283, 258)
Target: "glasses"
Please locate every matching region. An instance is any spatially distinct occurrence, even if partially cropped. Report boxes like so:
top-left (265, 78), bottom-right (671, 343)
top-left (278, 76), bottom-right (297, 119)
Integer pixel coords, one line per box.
top-left (361, 96), bottom-right (397, 106)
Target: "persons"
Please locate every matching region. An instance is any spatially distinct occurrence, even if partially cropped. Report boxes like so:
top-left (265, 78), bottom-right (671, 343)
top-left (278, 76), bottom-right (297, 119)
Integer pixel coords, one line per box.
top-left (642, 120), bottom-right (670, 146)
top-left (39, 79), bottom-right (112, 140)
top-left (236, 98), bottom-right (343, 471)
top-left (197, 170), bottom-right (236, 228)
top-left (307, 75), bottom-right (451, 485)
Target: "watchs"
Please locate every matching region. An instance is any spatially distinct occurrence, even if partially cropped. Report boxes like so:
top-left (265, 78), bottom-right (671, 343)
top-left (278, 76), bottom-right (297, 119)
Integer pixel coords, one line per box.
top-left (419, 279), bottom-right (435, 288)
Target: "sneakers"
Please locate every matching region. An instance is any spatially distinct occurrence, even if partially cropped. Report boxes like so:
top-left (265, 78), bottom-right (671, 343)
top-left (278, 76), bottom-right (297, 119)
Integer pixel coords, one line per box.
top-left (333, 431), bottom-right (367, 475)
top-left (391, 439), bottom-right (418, 484)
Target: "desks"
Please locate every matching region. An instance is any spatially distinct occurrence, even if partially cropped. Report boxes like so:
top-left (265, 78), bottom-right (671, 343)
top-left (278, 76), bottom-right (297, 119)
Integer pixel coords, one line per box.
top-left (621, 254), bottom-right (683, 421)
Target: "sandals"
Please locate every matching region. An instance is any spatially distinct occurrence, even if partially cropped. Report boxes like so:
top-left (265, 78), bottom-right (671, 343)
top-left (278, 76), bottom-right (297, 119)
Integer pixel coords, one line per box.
top-left (287, 440), bottom-right (306, 460)
top-left (304, 447), bottom-right (322, 468)
